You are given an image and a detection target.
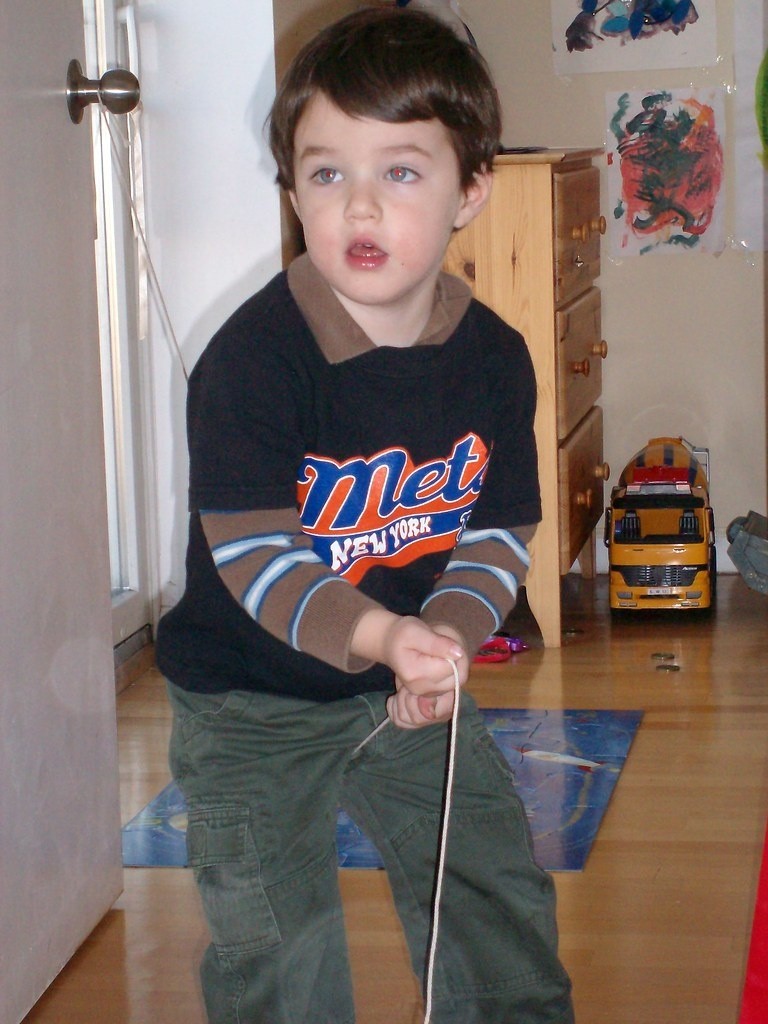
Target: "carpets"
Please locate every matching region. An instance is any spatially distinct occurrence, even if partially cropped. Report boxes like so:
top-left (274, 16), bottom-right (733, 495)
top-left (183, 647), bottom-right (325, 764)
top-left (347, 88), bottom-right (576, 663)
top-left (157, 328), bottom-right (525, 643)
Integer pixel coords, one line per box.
top-left (123, 707), bottom-right (648, 870)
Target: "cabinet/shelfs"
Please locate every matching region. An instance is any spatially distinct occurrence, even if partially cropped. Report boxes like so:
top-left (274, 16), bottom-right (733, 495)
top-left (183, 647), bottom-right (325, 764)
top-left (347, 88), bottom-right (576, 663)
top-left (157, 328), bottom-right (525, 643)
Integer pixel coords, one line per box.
top-left (417, 142), bottom-right (612, 652)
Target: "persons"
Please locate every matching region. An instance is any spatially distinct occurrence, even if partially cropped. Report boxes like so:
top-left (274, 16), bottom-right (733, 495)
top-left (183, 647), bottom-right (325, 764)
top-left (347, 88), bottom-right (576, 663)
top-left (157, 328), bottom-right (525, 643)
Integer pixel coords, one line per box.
top-left (155, 5), bottom-right (580, 1024)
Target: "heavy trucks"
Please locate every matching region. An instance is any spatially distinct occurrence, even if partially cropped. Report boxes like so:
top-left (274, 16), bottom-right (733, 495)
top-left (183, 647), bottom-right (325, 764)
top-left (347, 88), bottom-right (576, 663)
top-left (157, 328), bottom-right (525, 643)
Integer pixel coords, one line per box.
top-left (602, 437), bottom-right (717, 620)
